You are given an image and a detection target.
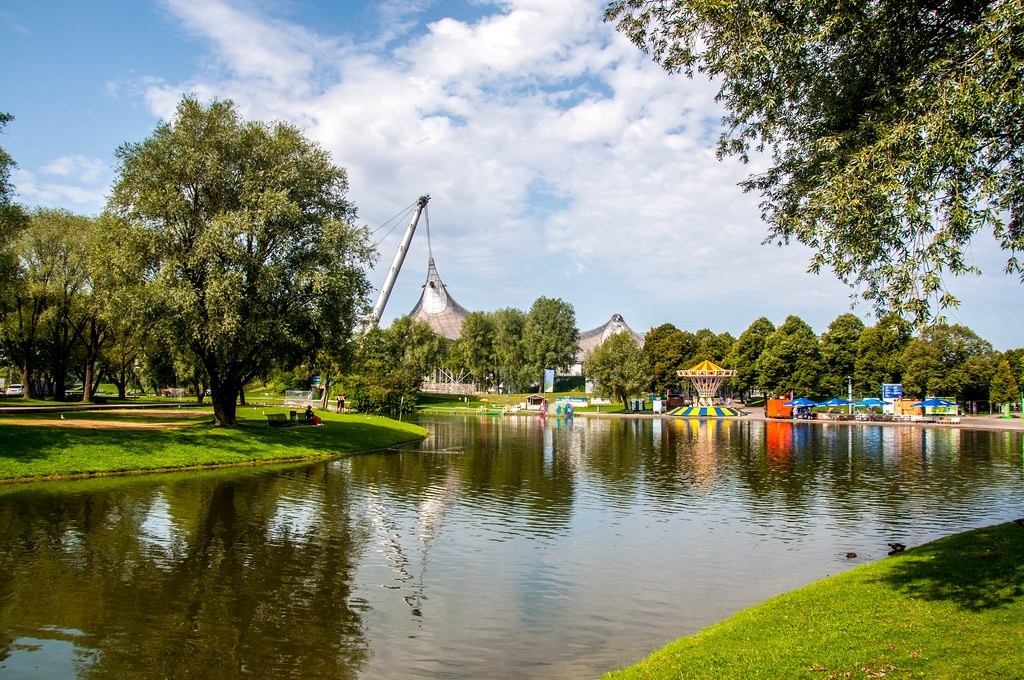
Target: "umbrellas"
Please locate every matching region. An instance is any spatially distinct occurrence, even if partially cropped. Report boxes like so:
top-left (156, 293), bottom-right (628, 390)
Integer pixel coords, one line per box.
top-left (817, 398), bottom-right (854, 407)
top-left (782, 398), bottom-right (817, 412)
top-left (854, 399), bottom-right (892, 406)
top-left (911, 398), bottom-right (960, 416)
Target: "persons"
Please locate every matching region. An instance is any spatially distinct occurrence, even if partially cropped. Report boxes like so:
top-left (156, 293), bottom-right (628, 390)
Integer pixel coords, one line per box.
top-left (867, 408), bottom-right (870, 414)
top-left (337, 394), bottom-right (344, 412)
top-left (828, 406), bottom-right (833, 413)
top-left (305, 405), bottom-right (324, 426)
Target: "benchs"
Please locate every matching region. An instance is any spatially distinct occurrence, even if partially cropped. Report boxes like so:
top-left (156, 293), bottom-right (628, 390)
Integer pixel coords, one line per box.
top-left (266, 414), bottom-right (290, 428)
top-left (296, 413), bottom-right (313, 425)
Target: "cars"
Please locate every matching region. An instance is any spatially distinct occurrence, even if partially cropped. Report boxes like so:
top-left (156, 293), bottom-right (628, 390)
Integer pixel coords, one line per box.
top-left (6, 383), bottom-right (25, 397)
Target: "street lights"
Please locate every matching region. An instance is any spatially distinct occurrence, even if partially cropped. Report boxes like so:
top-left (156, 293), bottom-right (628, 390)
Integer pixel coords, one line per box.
top-left (846, 374), bottom-right (854, 414)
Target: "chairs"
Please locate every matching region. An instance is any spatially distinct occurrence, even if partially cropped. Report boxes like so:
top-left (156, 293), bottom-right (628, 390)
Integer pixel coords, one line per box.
top-left (823, 412), bottom-right (961, 425)
